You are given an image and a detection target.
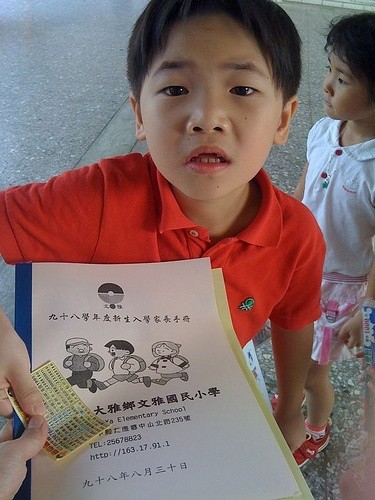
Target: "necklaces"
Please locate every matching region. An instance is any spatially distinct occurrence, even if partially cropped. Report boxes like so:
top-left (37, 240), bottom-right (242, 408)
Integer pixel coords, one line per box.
top-left (321, 124), bottom-right (373, 188)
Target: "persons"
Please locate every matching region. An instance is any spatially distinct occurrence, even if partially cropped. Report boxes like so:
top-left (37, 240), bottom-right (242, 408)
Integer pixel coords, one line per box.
top-left (271, 12), bottom-right (375, 467)
top-left (0, 0), bottom-right (327, 454)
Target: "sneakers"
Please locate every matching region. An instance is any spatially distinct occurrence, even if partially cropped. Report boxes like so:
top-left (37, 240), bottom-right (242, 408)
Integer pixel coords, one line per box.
top-left (293, 417), bottom-right (332, 467)
top-left (272, 389), bottom-right (306, 410)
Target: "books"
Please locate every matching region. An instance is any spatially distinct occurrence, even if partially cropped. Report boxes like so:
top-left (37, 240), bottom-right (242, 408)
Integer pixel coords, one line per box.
top-left (14, 257), bottom-right (313, 500)
